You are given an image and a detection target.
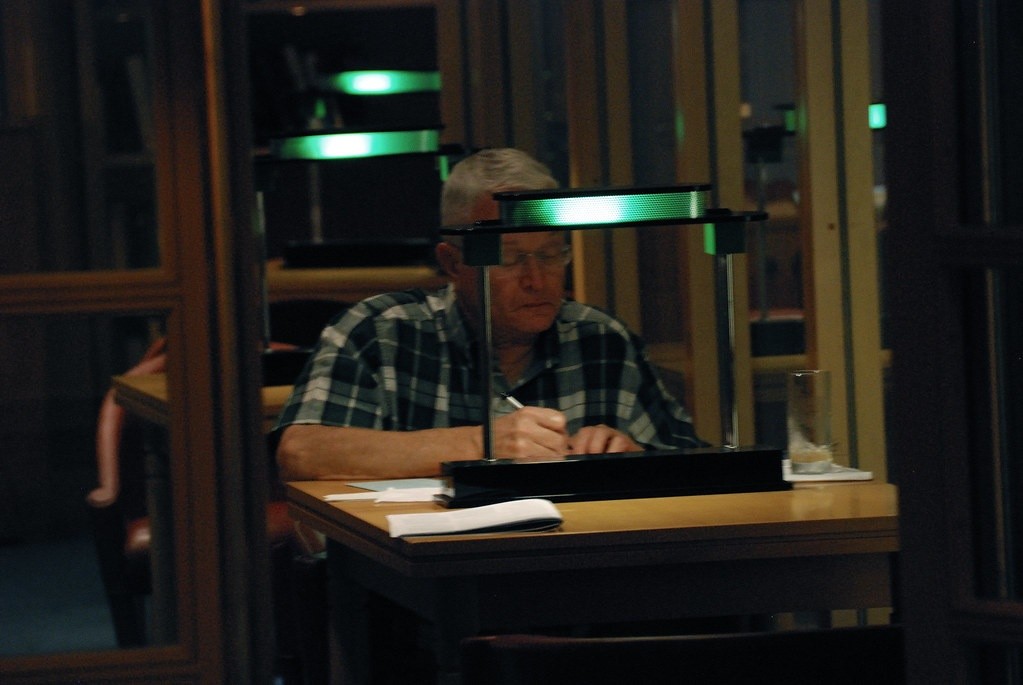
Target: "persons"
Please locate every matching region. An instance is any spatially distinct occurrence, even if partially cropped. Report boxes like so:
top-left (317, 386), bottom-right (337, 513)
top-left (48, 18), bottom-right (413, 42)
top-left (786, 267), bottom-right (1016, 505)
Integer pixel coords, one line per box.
top-left (276, 148), bottom-right (711, 481)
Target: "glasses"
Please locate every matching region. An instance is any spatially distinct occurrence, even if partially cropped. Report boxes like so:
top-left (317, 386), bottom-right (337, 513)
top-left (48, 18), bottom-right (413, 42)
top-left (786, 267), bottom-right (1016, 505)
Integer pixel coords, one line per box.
top-left (491, 242), bottom-right (573, 280)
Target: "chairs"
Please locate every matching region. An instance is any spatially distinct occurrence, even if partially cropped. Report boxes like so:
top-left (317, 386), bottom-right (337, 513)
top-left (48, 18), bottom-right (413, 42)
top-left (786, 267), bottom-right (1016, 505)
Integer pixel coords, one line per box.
top-left (89, 337), bottom-right (311, 648)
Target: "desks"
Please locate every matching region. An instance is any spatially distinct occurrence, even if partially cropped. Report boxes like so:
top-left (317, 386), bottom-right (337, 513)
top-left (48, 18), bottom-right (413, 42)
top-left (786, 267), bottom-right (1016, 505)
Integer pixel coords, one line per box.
top-left (282, 477), bottom-right (902, 685)
top-left (111, 371), bottom-right (297, 639)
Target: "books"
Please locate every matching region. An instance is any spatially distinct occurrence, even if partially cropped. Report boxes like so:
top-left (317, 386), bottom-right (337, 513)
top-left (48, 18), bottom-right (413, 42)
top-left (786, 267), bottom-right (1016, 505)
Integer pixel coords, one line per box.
top-left (387, 499), bottom-right (565, 537)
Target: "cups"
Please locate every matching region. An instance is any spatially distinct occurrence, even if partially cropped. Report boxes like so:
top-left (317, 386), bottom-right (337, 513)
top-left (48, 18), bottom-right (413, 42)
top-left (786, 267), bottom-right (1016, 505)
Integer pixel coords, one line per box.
top-left (782, 368), bottom-right (836, 475)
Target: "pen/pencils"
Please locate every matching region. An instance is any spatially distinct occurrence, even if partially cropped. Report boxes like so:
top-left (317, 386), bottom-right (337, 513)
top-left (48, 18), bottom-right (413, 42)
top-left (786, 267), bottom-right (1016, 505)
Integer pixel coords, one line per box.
top-left (499, 390), bottom-right (574, 451)
